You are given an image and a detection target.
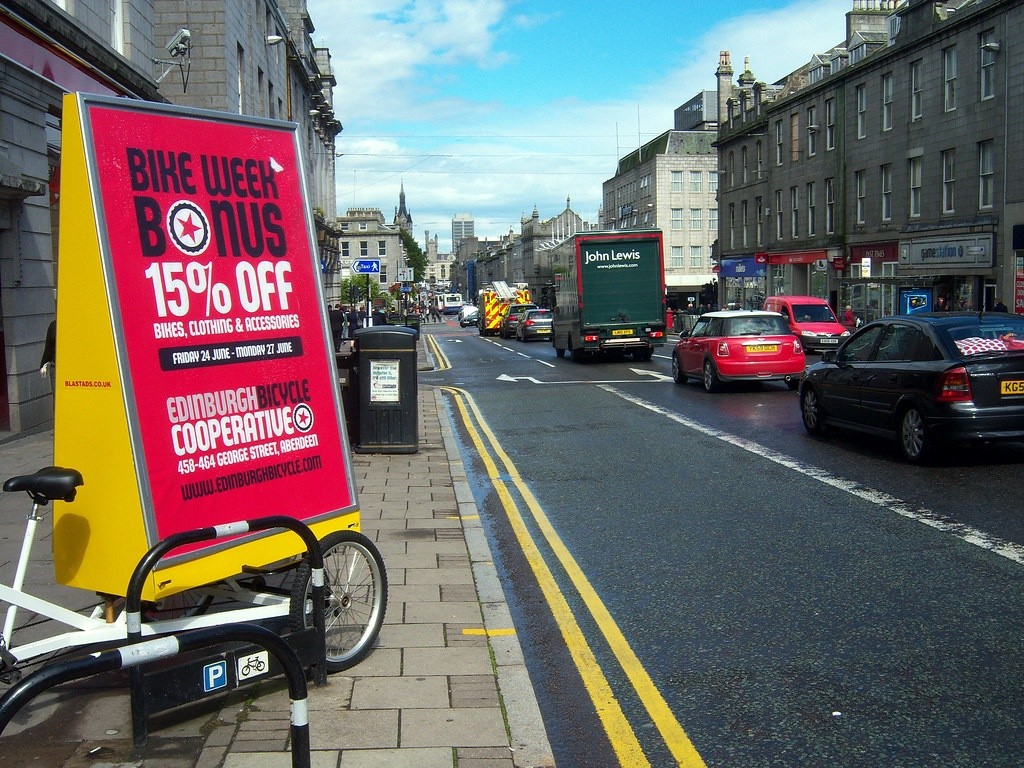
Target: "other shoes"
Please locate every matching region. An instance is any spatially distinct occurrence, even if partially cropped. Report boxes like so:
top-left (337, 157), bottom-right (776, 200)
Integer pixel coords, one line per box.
top-left (350, 346), bottom-right (353, 352)
top-left (342, 341), bottom-right (346, 345)
top-left (335, 349), bottom-right (342, 353)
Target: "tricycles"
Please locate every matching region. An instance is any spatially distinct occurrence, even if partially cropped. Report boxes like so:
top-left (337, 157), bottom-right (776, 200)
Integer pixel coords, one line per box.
top-left (0, 465), bottom-right (388, 687)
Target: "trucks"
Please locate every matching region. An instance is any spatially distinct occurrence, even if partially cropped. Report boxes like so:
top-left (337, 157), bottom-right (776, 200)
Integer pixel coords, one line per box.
top-left (547, 227), bottom-right (668, 365)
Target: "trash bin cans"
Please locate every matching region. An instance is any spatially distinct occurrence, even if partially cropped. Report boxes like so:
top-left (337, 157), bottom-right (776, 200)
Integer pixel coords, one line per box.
top-left (405, 313), bottom-right (421, 341)
top-left (354, 323), bottom-right (421, 455)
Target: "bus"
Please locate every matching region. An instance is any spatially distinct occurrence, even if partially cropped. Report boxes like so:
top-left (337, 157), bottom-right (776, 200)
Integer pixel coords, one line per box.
top-left (443, 294), bottom-right (462, 315)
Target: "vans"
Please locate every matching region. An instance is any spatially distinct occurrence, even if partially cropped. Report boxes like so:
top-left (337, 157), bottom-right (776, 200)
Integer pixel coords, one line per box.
top-left (762, 295), bottom-right (851, 353)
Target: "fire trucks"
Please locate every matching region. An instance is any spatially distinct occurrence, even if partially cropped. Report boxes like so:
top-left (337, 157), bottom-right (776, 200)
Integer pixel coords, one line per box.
top-left (475, 281), bottom-right (530, 336)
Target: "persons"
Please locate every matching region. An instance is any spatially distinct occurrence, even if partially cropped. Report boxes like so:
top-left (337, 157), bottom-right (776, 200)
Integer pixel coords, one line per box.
top-left (687, 302), bottom-right (745, 315)
top-left (844, 306), bottom-right (856, 326)
top-left (409, 306), bottom-right (436, 323)
top-left (327, 303), bottom-right (386, 353)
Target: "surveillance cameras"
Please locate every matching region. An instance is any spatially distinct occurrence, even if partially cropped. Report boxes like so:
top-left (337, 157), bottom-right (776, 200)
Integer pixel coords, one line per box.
top-left (165, 28), bottom-right (190, 53)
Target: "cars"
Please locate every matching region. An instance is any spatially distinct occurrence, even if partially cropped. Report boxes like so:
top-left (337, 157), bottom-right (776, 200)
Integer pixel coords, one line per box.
top-left (515, 309), bottom-right (553, 343)
top-left (458, 305), bottom-right (476, 321)
top-left (672, 310), bottom-right (807, 393)
top-left (795, 310), bottom-right (1024, 466)
top-left (460, 310), bottom-right (479, 329)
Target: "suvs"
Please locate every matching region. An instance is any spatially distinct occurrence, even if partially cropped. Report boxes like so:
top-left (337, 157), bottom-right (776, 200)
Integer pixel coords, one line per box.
top-left (499, 303), bottom-right (539, 340)
